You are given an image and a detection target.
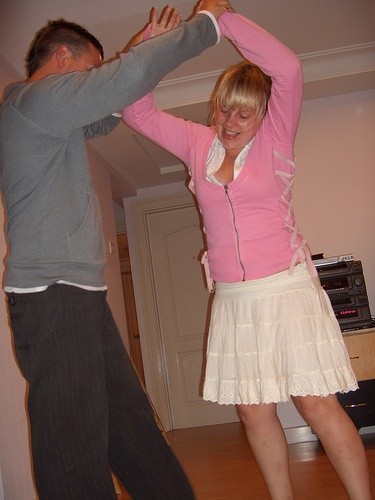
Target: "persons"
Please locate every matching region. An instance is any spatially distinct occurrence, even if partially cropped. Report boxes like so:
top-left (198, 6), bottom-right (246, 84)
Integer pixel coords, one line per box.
top-left (0, 2), bottom-right (234, 500)
top-left (122, 0), bottom-right (374, 500)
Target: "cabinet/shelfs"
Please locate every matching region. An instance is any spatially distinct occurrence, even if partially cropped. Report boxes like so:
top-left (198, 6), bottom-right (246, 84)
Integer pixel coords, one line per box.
top-left (309, 327), bottom-right (375, 442)
